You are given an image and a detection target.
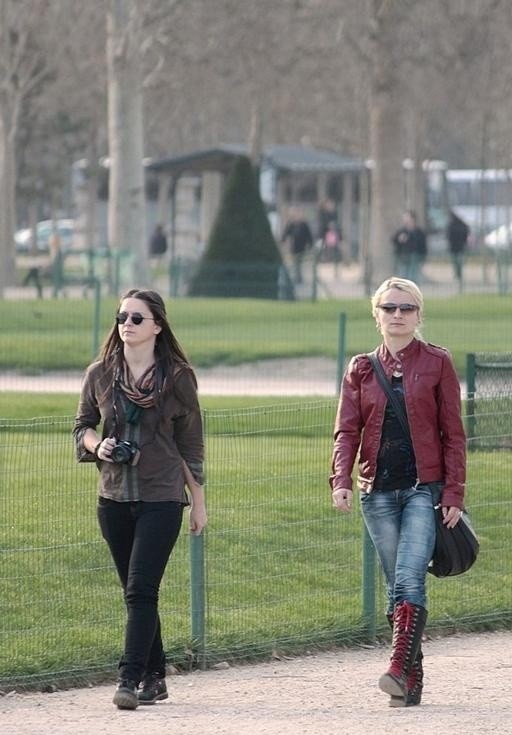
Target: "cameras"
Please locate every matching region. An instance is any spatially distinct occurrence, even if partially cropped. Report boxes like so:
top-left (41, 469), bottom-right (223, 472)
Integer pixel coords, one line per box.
top-left (110, 440), bottom-right (141, 467)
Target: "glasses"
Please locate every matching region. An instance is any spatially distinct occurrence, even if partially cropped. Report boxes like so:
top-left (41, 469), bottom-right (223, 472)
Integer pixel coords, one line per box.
top-left (376, 303), bottom-right (420, 313)
top-left (116, 312), bottom-right (155, 326)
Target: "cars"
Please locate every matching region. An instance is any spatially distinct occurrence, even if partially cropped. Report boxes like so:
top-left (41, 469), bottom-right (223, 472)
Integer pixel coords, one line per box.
top-left (485, 218), bottom-right (511, 258)
top-left (14, 217), bottom-right (76, 256)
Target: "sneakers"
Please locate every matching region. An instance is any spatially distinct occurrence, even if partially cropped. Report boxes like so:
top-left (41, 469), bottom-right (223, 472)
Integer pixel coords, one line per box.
top-left (135, 676), bottom-right (169, 705)
top-left (112, 676), bottom-right (139, 711)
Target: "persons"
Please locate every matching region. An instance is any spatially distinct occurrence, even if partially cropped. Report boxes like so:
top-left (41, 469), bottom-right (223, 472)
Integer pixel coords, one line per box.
top-left (148, 226), bottom-right (167, 258)
top-left (390, 207), bottom-right (427, 280)
top-left (327, 276), bottom-right (466, 708)
top-left (71, 288), bottom-right (207, 708)
top-left (314, 196), bottom-right (344, 287)
top-left (442, 210), bottom-right (470, 279)
top-left (20, 242), bottom-right (43, 299)
top-left (282, 206), bottom-right (314, 287)
top-left (48, 233), bottom-right (71, 297)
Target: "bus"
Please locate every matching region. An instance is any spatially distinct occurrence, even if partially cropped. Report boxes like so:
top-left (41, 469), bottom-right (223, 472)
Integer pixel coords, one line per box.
top-left (446, 169), bottom-right (511, 245)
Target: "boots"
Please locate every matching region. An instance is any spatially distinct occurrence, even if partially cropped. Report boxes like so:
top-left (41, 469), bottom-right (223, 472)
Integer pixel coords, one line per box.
top-left (378, 599), bottom-right (429, 708)
top-left (385, 610), bottom-right (425, 708)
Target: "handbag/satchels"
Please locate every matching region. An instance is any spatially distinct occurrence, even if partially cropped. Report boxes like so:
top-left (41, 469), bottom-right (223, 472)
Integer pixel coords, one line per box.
top-left (426, 486), bottom-right (481, 579)
